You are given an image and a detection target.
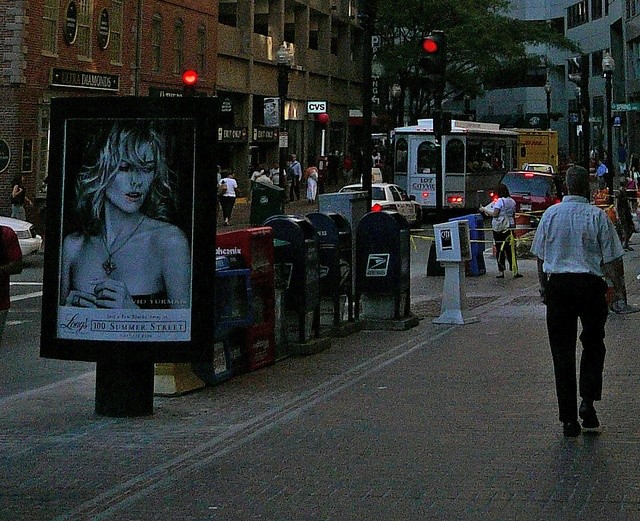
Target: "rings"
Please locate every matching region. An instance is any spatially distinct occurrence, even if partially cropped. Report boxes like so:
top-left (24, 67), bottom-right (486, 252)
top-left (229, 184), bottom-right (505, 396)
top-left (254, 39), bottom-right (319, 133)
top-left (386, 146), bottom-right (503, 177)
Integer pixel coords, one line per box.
top-left (77, 297), bottom-right (82, 306)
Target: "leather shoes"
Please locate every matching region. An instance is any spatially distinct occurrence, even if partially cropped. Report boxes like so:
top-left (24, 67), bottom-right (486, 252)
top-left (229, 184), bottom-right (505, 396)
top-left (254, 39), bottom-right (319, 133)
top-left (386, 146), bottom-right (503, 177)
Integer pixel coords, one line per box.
top-left (496, 274), bottom-right (504, 278)
top-left (563, 422), bottom-right (581, 438)
top-left (514, 273), bottom-right (524, 278)
top-left (579, 402), bottom-right (599, 428)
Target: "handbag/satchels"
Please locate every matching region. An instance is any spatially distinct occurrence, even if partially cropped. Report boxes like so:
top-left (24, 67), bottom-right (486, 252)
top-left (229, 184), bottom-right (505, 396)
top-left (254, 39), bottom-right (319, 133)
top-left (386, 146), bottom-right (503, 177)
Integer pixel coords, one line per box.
top-left (492, 215), bottom-right (510, 232)
top-left (10, 190), bottom-right (25, 205)
top-left (311, 172), bottom-right (318, 180)
top-left (217, 185), bottom-right (227, 197)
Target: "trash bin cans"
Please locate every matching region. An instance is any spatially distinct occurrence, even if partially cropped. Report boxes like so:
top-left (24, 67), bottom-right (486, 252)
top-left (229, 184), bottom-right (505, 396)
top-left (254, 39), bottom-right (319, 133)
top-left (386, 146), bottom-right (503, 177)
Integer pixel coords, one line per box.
top-left (264, 211), bottom-right (330, 354)
top-left (247, 180), bottom-right (285, 224)
top-left (351, 207), bottom-right (420, 331)
top-left (310, 208), bottom-right (357, 338)
top-left (192, 256), bottom-right (250, 385)
top-left (218, 227), bottom-right (280, 364)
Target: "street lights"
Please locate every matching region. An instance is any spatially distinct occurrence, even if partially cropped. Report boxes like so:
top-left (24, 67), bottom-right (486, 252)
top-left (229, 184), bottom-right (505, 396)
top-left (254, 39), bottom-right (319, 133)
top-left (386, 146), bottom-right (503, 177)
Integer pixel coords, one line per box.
top-left (602, 52), bottom-right (615, 204)
top-left (275, 46), bottom-right (291, 214)
top-left (545, 79), bottom-right (553, 131)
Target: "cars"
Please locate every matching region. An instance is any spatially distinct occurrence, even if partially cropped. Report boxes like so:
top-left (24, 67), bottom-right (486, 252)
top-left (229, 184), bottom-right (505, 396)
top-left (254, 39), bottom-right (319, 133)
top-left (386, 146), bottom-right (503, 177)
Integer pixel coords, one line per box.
top-left (491, 164), bottom-right (560, 224)
top-left (339, 182), bottom-right (422, 226)
top-left (0, 216), bottom-right (44, 258)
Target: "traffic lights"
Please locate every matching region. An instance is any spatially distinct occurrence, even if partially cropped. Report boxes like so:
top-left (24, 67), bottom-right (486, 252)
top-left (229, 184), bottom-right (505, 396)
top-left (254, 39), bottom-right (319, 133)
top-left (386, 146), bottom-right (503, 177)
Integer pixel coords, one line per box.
top-left (182, 71), bottom-right (198, 97)
top-left (419, 36), bottom-right (447, 92)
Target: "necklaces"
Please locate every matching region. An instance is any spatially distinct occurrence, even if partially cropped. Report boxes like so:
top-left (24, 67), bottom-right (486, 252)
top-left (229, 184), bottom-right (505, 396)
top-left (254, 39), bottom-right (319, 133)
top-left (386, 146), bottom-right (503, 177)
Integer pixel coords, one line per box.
top-left (100, 214), bottom-right (146, 277)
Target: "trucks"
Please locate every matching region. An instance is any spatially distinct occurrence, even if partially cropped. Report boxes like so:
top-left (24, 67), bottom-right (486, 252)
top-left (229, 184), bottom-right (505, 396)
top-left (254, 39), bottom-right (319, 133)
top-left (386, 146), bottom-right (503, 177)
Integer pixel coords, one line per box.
top-left (504, 128), bottom-right (558, 178)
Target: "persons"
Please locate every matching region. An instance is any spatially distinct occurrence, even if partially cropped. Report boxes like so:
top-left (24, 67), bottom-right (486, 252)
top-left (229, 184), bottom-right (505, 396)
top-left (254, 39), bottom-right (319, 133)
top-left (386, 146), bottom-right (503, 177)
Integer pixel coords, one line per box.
top-left (305, 160), bottom-right (318, 205)
top-left (287, 154), bottom-right (303, 202)
top-left (218, 168), bottom-right (238, 226)
top-left (595, 158), bottom-right (608, 189)
top-left (327, 141), bottom-right (407, 187)
top-left (250, 162), bottom-right (286, 189)
top-left (0, 225), bottom-right (23, 342)
top-left (615, 176), bottom-right (636, 252)
top-left (617, 141), bottom-right (640, 182)
top-left (528, 164), bottom-right (627, 437)
top-left (217, 166), bottom-right (222, 226)
top-left (478, 182), bottom-right (523, 279)
top-left (8, 172), bottom-right (34, 221)
top-left (285, 160), bottom-right (295, 203)
top-left (59, 119), bottom-right (190, 309)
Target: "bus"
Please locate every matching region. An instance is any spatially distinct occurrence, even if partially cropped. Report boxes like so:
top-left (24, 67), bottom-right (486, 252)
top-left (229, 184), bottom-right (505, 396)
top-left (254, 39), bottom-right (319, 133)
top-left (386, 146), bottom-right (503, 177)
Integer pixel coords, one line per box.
top-left (393, 119), bottom-right (526, 221)
